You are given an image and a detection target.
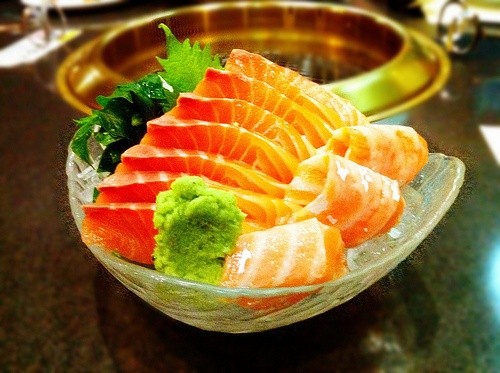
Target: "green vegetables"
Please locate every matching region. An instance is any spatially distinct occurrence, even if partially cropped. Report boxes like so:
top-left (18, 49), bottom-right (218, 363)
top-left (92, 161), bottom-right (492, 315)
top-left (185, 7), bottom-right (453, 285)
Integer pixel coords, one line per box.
top-left (68, 22), bottom-right (224, 204)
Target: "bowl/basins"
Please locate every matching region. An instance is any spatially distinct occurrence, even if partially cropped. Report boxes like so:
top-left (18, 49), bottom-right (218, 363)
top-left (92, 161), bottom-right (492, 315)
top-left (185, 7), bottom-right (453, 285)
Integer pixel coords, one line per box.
top-left (65, 117), bottom-right (465, 332)
